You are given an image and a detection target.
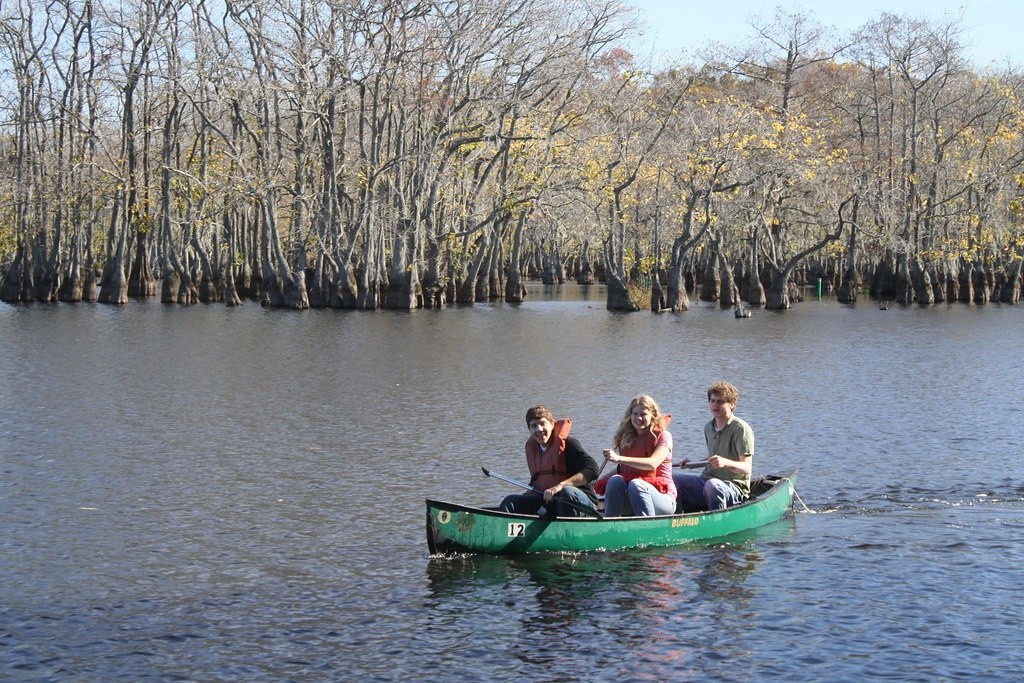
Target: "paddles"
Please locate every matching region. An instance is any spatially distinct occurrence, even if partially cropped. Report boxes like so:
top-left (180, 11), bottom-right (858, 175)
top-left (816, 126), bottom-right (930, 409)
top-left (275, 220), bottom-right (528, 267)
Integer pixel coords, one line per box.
top-left (672, 460), bottom-right (710, 466)
top-left (592, 442), bottom-right (619, 484)
top-left (481, 466), bottom-right (604, 521)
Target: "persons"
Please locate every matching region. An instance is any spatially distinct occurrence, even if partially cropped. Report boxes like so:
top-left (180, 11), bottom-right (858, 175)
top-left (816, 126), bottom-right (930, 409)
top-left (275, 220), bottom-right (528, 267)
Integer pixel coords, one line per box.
top-left (673, 382), bottom-right (755, 513)
top-left (596, 395), bottom-right (678, 517)
top-left (500, 406), bottom-right (599, 517)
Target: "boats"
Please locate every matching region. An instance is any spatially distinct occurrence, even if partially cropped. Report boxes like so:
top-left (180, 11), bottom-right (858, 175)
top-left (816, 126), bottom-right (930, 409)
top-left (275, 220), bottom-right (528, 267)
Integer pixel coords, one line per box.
top-left (426, 466), bottom-right (798, 553)
